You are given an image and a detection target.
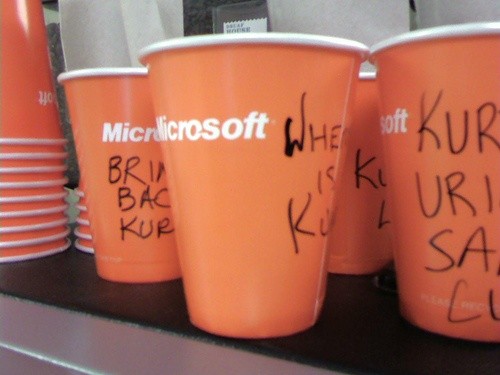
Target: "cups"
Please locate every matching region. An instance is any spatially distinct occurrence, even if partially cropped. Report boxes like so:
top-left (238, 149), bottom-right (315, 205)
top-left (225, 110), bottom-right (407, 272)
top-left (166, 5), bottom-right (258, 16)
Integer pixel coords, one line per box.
top-left (139, 33), bottom-right (370, 339)
top-left (367, 21), bottom-right (499, 343)
top-left (56, 68), bottom-right (181, 283)
top-left (325, 71), bottom-right (392, 275)
top-left (0, 0), bottom-right (94, 263)
